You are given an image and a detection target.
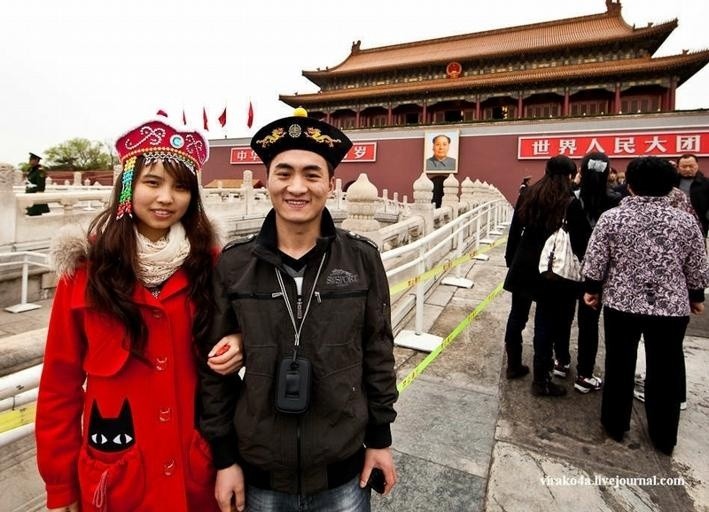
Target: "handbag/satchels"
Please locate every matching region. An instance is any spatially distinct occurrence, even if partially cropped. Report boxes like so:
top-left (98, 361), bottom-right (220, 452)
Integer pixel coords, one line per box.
top-left (538, 222), bottom-right (586, 288)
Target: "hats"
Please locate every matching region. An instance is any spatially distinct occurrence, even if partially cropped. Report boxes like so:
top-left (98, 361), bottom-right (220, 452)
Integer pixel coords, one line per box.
top-left (110, 114), bottom-right (210, 221)
top-left (250, 116), bottom-right (354, 169)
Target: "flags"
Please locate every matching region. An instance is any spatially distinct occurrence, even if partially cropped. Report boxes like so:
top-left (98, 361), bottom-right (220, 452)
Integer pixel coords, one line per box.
top-left (247, 102), bottom-right (254, 130)
top-left (217, 107), bottom-right (227, 128)
top-left (203, 107), bottom-right (211, 133)
top-left (182, 109), bottom-right (187, 125)
top-left (157, 110), bottom-right (169, 119)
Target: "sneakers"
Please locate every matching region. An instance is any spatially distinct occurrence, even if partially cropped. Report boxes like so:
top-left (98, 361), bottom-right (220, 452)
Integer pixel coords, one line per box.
top-left (506, 343), bottom-right (687, 410)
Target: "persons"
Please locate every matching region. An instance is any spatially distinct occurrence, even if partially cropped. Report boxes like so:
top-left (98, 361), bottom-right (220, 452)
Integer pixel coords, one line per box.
top-left (24, 151), bottom-right (51, 216)
top-left (501, 152), bottom-right (708, 457)
top-left (426, 134), bottom-right (456, 170)
top-left (34, 109), bottom-right (245, 512)
top-left (195, 105), bottom-right (400, 511)
